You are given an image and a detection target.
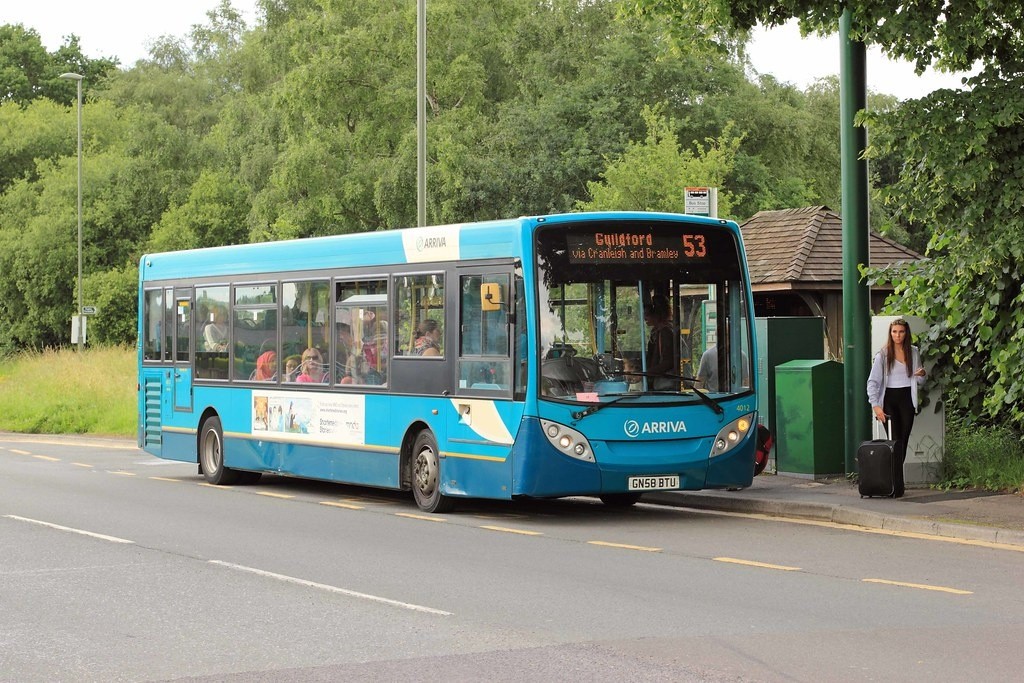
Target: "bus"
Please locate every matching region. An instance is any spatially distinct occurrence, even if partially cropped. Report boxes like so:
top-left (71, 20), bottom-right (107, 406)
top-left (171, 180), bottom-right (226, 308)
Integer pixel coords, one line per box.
top-left (137, 211), bottom-right (759, 513)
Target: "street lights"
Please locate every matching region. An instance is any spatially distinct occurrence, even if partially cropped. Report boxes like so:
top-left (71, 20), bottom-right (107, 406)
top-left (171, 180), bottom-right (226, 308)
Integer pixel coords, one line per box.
top-left (59, 73), bottom-right (82, 352)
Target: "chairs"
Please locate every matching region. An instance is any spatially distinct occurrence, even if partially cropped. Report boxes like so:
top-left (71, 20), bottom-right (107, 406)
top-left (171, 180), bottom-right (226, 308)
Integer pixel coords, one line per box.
top-left (287, 370), bottom-right (301, 382)
top-left (322, 369), bottom-right (346, 384)
top-left (247, 368), bottom-right (258, 380)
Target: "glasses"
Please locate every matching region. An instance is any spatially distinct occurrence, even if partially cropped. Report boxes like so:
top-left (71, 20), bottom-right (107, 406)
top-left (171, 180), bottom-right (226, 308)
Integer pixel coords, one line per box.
top-left (891, 320), bottom-right (906, 325)
top-left (305, 356), bottom-right (319, 360)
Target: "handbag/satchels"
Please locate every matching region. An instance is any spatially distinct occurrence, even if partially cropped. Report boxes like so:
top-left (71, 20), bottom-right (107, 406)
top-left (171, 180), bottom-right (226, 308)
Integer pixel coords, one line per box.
top-left (653, 325), bottom-right (694, 390)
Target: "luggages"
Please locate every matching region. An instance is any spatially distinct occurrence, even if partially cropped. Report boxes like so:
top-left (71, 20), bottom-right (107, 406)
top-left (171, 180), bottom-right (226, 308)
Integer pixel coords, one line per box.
top-left (858, 415), bottom-right (896, 499)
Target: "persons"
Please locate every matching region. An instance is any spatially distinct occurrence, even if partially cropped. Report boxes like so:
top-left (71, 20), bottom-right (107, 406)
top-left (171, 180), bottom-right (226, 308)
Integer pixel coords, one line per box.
top-left (695, 323), bottom-right (749, 390)
top-left (406, 319), bottom-right (440, 356)
top-left (645, 295), bottom-right (693, 393)
top-left (623, 359), bottom-right (642, 392)
top-left (204, 305), bottom-right (229, 351)
top-left (867, 319), bottom-right (927, 501)
top-left (252, 311), bottom-right (388, 385)
